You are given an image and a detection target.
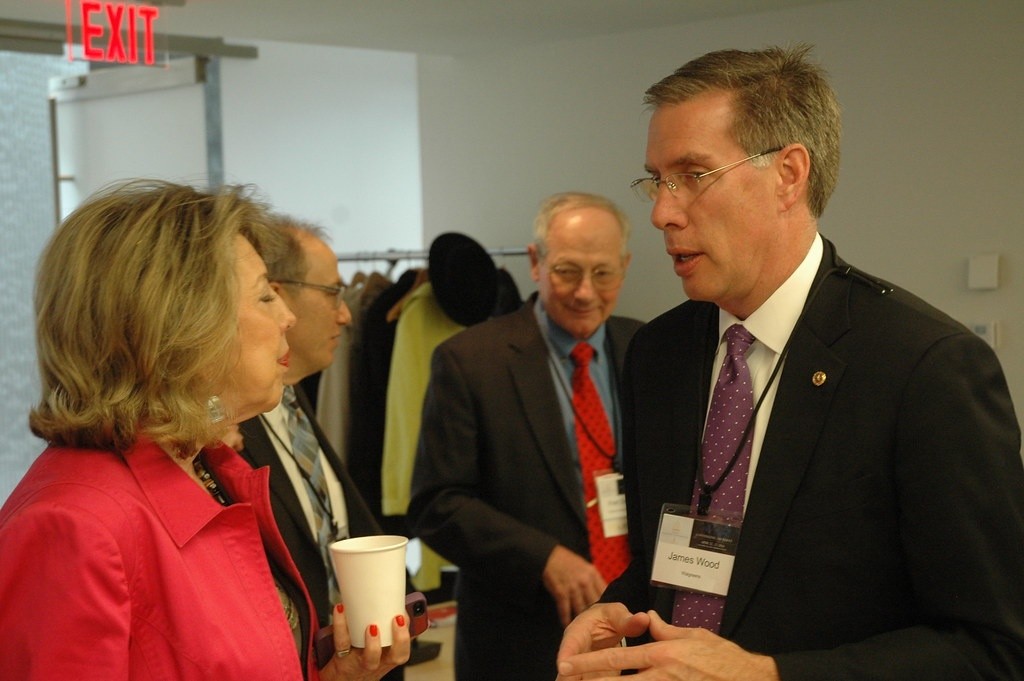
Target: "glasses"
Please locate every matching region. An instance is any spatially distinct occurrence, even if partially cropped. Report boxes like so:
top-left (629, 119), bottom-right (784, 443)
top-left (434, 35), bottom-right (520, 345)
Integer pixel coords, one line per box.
top-left (629, 146), bottom-right (786, 202)
top-left (271, 278), bottom-right (347, 311)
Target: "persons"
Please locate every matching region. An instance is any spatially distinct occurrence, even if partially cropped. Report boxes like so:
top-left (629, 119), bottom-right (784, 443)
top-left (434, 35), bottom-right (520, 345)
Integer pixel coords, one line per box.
top-left (410, 191), bottom-right (649, 681)
top-left (556, 45), bottom-right (1024, 681)
top-left (247, 217), bottom-right (406, 681)
top-left (0, 179), bottom-right (412, 681)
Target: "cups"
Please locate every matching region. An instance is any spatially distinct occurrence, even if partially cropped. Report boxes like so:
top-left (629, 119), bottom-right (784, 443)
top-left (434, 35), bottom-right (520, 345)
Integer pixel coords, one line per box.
top-left (330, 534), bottom-right (408, 648)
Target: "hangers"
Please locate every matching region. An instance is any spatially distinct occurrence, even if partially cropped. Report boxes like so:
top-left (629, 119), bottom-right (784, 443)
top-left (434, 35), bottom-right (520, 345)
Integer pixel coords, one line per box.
top-left (338, 248), bottom-right (428, 323)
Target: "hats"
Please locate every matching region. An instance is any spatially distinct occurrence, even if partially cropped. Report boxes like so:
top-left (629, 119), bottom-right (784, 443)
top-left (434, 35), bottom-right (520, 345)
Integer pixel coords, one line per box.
top-left (426, 230), bottom-right (501, 328)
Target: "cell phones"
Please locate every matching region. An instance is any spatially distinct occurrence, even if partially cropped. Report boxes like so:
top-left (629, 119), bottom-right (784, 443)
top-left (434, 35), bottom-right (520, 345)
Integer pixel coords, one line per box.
top-left (315, 592), bottom-right (428, 671)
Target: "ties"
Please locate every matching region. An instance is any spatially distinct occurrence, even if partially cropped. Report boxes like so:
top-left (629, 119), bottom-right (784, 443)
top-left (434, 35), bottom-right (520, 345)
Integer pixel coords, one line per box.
top-left (568, 340), bottom-right (630, 585)
top-left (281, 383), bottom-right (340, 608)
top-left (668, 324), bottom-right (757, 639)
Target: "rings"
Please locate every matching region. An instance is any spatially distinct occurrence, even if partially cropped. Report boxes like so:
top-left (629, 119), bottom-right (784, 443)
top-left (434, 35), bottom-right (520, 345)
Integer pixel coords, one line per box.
top-left (335, 648), bottom-right (351, 658)
